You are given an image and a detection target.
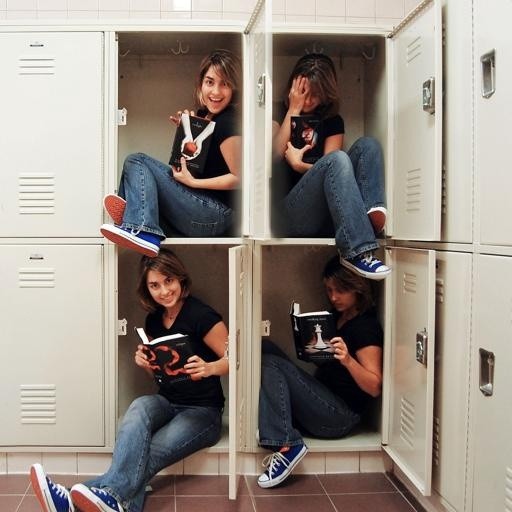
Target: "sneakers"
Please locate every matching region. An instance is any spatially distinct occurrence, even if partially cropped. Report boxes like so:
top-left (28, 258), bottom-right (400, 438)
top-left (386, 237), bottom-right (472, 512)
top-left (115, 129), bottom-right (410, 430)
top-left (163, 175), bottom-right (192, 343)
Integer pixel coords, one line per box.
top-left (368, 207), bottom-right (385, 234)
top-left (258, 444), bottom-right (309, 488)
top-left (101, 225), bottom-right (161, 257)
top-left (104, 194), bottom-right (126, 225)
top-left (71, 485), bottom-right (125, 512)
top-left (31, 463), bottom-right (75, 512)
top-left (341, 250), bottom-right (392, 281)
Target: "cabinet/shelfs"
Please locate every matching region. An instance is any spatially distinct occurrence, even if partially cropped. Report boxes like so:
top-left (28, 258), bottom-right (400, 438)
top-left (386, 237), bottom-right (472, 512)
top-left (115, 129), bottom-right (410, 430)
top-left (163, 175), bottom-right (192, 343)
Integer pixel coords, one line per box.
top-left (394, 0), bottom-right (511, 511)
top-left (1, 19), bottom-right (114, 455)
top-left (113, 1), bottom-right (447, 502)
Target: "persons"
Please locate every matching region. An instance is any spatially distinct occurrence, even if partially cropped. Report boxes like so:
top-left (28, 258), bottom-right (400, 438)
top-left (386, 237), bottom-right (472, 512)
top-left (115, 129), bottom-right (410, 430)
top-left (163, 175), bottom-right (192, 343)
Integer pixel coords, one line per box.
top-left (30, 249), bottom-right (229, 511)
top-left (99, 48), bottom-right (242, 258)
top-left (257, 257), bottom-right (383, 487)
top-left (273, 53), bottom-right (391, 281)
